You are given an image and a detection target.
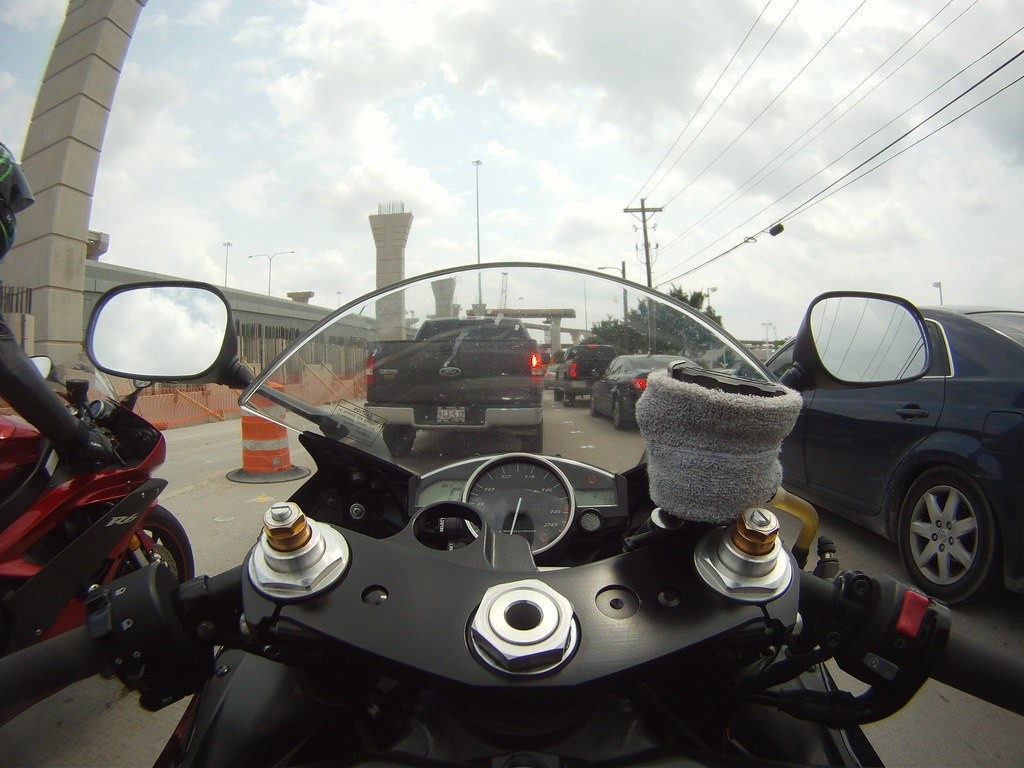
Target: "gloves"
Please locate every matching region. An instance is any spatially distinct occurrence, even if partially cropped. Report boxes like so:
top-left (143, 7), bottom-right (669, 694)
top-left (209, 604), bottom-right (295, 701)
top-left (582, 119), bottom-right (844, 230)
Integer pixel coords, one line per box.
top-left (69, 430), bottom-right (111, 475)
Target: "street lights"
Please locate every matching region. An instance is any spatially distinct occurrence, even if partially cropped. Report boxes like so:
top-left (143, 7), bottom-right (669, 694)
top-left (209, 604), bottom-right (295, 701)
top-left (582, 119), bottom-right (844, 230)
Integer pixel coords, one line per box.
top-left (598, 262), bottom-right (628, 321)
top-left (248, 251), bottom-right (295, 296)
top-left (932, 282), bottom-right (942, 304)
top-left (707, 287), bottom-right (717, 306)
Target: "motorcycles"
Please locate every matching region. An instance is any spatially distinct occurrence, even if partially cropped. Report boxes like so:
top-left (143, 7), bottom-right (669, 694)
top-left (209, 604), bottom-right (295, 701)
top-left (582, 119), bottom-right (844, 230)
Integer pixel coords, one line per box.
top-left (1, 355), bottom-right (195, 651)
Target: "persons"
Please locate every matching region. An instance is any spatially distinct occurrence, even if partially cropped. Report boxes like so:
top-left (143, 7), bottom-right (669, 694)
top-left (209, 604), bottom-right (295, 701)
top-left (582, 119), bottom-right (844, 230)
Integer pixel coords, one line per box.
top-left (0, 141), bottom-right (91, 470)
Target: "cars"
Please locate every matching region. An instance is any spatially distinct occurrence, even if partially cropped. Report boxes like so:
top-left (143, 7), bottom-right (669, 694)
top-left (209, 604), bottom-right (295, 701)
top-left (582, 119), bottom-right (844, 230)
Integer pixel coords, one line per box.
top-left (543, 366), bottom-right (559, 390)
top-left (589, 355), bottom-right (705, 430)
top-left (761, 305), bottom-right (1024, 611)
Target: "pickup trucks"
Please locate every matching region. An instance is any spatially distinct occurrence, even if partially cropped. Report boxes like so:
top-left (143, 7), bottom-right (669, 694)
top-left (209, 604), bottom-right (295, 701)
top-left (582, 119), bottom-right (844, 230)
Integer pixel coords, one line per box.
top-left (553, 343), bottom-right (619, 407)
top-left (366, 316), bottom-right (550, 458)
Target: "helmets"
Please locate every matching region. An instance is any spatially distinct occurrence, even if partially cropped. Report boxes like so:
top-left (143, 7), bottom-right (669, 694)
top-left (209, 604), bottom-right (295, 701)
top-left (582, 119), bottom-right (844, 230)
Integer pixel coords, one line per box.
top-left (0, 142), bottom-right (34, 262)
